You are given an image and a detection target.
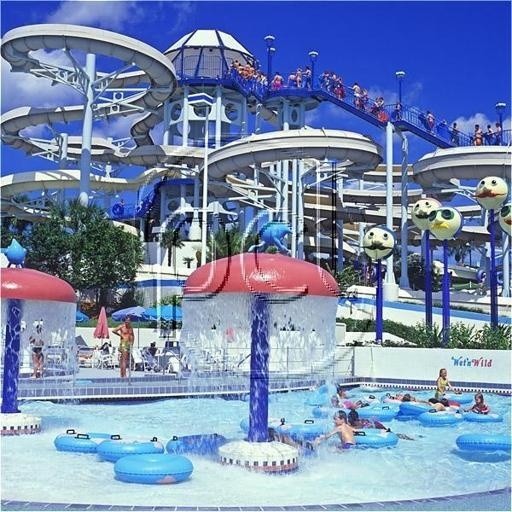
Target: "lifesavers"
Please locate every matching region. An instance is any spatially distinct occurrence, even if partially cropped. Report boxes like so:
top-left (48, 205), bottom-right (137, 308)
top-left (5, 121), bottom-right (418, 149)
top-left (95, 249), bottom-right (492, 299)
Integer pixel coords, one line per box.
top-left (97, 435), bottom-right (164, 462)
top-left (240, 418), bottom-right (287, 433)
top-left (275, 420), bottom-right (334, 443)
top-left (311, 393), bottom-right (504, 428)
top-left (114, 454), bottom-right (193, 484)
top-left (457, 434), bottom-right (511, 451)
top-left (326, 429), bottom-right (398, 450)
top-left (54, 430), bottom-right (112, 453)
top-left (166, 434), bottom-right (225, 455)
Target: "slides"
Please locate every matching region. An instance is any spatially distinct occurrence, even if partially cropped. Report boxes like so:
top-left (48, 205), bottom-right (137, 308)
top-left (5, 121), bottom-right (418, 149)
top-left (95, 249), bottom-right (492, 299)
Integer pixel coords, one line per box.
top-left (1, 24), bottom-right (511, 282)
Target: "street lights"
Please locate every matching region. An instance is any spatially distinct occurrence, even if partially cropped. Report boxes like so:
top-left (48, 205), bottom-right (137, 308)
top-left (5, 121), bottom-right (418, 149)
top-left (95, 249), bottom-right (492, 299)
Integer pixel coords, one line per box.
top-left (395, 71), bottom-right (405, 104)
top-left (495, 102), bottom-right (506, 145)
top-left (308, 51), bottom-right (318, 88)
top-left (264, 34), bottom-right (276, 85)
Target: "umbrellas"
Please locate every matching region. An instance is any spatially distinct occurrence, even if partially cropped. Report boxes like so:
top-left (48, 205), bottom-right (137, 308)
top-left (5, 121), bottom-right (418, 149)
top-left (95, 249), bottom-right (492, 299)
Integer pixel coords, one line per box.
top-left (111, 305), bottom-right (147, 350)
top-left (145, 304), bottom-right (184, 347)
top-left (74, 311), bottom-right (90, 322)
top-left (93, 306), bottom-right (109, 345)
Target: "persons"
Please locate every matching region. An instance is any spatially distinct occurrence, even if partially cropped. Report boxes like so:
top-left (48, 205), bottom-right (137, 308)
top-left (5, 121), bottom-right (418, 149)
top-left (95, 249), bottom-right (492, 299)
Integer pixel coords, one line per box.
top-left (494, 123), bottom-right (502, 145)
top-left (453, 123), bottom-right (459, 144)
top-left (426, 111), bottom-right (436, 130)
top-left (474, 124), bottom-right (483, 146)
top-left (316, 411), bottom-right (356, 450)
top-left (395, 100), bottom-right (402, 119)
top-left (112, 318), bottom-right (135, 378)
top-left (435, 368), bottom-right (460, 400)
top-left (334, 387), bottom-right (488, 415)
top-left (487, 124), bottom-right (494, 145)
top-left (29, 325), bottom-right (45, 379)
top-left (148, 342), bottom-right (158, 355)
top-left (232, 58), bottom-right (385, 116)
